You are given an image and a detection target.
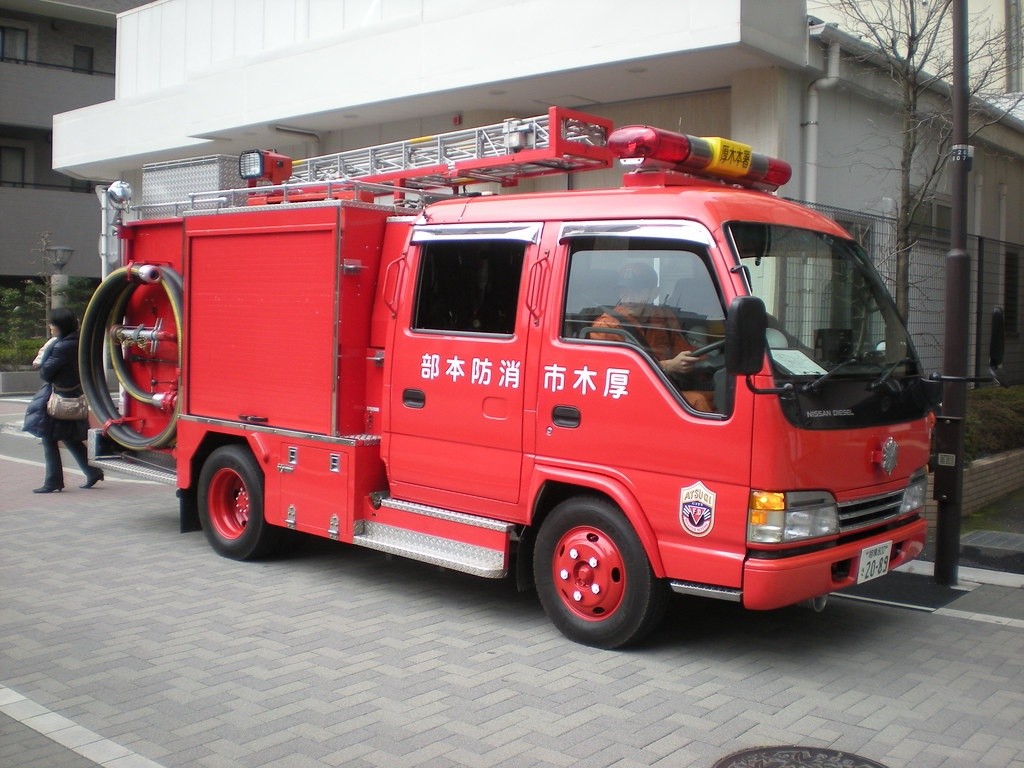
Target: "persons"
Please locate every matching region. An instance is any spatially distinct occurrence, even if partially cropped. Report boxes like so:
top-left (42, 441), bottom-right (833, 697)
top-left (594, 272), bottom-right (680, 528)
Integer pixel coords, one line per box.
top-left (20, 308), bottom-right (105, 494)
top-left (29, 315), bottom-right (81, 371)
top-left (590, 261), bottom-right (717, 417)
top-left (685, 264), bottom-right (818, 361)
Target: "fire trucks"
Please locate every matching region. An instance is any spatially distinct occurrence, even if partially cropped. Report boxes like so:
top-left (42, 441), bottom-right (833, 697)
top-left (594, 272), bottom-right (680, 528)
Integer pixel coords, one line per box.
top-left (78, 103), bottom-right (937, 648)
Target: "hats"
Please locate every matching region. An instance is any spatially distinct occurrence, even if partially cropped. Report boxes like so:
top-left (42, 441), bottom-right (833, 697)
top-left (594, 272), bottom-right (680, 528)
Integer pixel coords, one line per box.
top-left (613, 263), bottom-right (658, 290)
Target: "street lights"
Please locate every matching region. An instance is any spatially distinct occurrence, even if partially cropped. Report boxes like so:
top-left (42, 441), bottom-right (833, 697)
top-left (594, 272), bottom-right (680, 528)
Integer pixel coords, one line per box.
top-left (43, 246), bottom-right (75, 307)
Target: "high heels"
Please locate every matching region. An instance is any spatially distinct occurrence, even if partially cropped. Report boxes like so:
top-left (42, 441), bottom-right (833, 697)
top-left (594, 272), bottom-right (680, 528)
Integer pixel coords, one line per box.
top-left (33, 486), bottom-right (64, 493)
top-left (80, 473), bottom-right (104, 488)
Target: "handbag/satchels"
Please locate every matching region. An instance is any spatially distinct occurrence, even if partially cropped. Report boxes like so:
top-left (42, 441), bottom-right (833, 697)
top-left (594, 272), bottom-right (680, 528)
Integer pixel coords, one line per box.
top-left (47, 392), bottom-right (88, 420)
